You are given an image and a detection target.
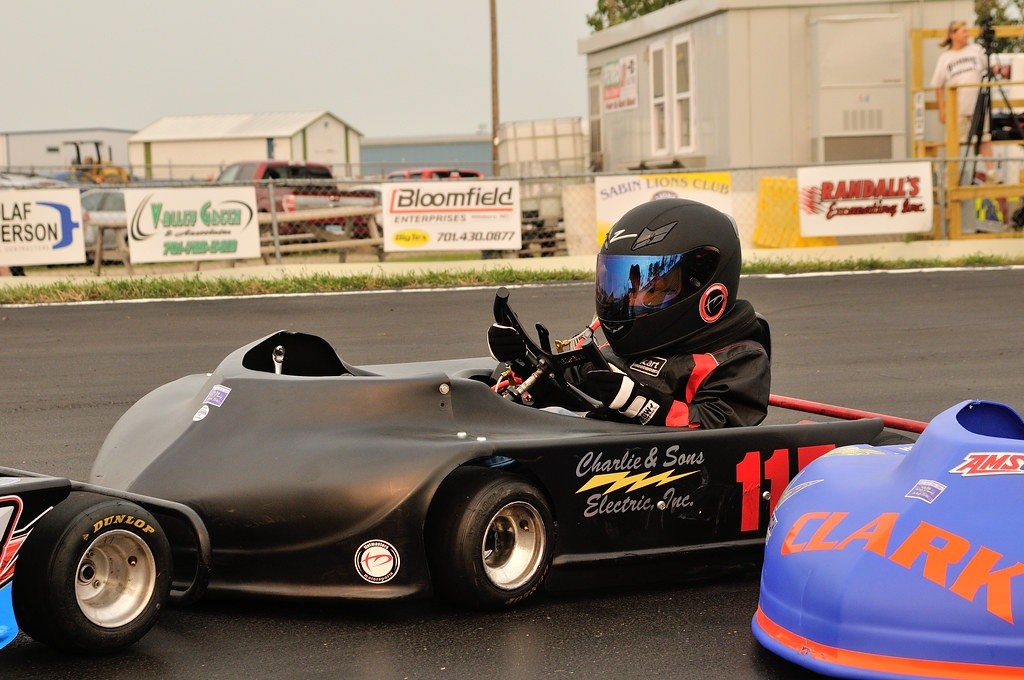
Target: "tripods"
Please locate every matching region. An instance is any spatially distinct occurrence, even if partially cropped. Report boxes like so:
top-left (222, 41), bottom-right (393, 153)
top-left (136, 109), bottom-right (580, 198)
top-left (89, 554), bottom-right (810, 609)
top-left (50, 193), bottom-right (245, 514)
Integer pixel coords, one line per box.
top-left (958, 44), bottom-right (1024, 187)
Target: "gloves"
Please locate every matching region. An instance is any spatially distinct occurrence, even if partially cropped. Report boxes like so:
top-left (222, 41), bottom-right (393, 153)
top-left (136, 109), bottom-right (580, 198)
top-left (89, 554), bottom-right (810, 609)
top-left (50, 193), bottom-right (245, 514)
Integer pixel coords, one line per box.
top-left (587, 362), bottom-right (675, 427)
top-left (487, 321), bottom-right (528, 375)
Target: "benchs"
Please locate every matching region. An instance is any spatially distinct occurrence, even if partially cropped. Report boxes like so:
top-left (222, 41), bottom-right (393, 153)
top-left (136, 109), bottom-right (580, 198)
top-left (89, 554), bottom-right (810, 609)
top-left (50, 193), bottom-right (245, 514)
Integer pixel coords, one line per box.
top-left (91, 204), bottom-right (384, 275)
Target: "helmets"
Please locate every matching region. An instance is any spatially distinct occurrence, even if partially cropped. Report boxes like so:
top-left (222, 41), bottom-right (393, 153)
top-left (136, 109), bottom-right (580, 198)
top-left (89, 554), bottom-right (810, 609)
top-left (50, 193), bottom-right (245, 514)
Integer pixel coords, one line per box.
top-left (594, 198), bottom-right (743, 357)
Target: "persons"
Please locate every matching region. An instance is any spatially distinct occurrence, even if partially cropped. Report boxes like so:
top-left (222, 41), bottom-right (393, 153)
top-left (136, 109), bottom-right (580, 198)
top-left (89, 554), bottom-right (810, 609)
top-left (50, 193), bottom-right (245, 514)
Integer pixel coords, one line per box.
top-left (929, 20), bottom-right (1010, 186)
top-left (535, 198), bottom-right (770, 430)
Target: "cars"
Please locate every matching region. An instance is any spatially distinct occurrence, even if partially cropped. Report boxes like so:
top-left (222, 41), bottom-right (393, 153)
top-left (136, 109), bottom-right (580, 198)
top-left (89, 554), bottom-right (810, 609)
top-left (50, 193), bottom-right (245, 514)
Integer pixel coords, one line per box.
top-left (0, 171), bottom-right (208, 263)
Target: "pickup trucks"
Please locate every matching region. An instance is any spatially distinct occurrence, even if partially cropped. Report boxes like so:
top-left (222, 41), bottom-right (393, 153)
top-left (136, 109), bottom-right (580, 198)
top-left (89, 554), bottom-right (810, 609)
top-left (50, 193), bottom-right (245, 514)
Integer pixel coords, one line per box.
top-left (211, 161), bottom-right (374, 238)
